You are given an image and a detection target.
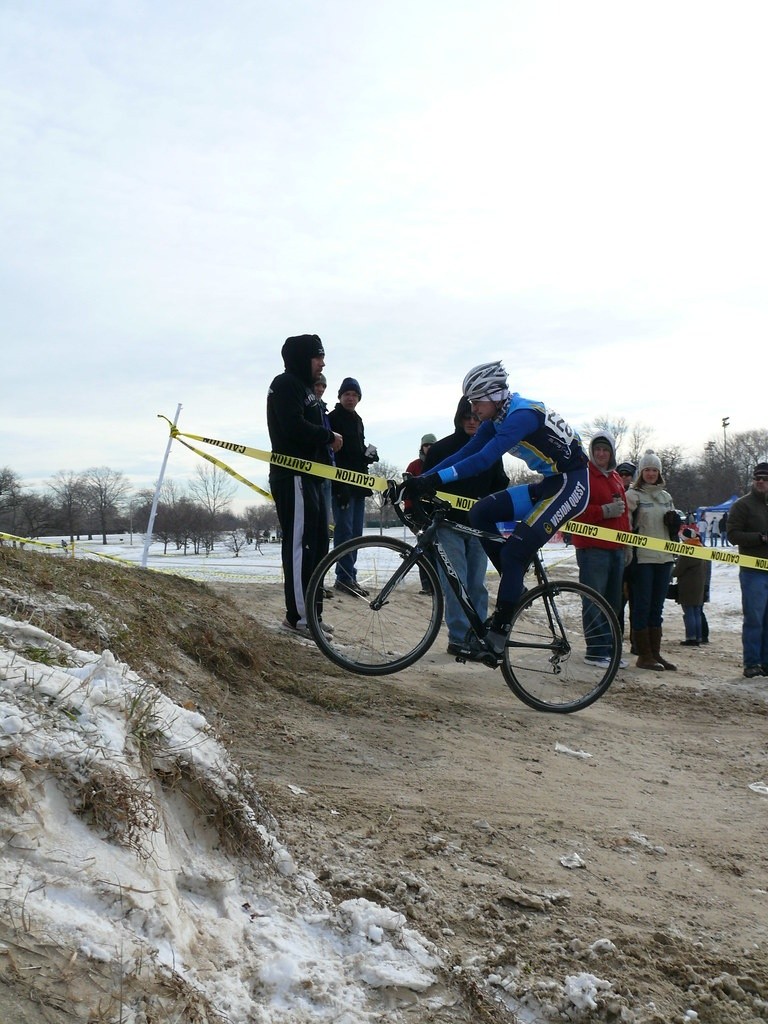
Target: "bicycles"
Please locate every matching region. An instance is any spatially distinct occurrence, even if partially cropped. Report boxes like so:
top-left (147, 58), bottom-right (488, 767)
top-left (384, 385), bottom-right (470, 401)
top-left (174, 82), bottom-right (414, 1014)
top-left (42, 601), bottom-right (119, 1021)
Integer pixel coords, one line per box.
top-left (306, 473), bottom-right (621, 714)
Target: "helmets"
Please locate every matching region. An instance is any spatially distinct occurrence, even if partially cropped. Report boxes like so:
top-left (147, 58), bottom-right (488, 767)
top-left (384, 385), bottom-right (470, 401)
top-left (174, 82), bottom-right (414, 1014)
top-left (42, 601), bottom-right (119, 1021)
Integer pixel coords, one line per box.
top-left (462, 360), bottom-right (509, 402)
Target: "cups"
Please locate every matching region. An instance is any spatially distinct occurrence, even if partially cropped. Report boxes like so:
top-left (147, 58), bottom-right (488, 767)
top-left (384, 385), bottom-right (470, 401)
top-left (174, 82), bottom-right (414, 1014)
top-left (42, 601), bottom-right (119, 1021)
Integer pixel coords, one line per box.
top-left (364, 444), bottom-right (377, 457)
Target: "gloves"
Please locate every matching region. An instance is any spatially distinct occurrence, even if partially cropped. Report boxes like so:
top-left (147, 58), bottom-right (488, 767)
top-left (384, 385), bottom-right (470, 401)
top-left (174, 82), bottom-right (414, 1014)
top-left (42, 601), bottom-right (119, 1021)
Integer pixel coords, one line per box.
top-left (624, 548), bottom-right (633, 567)
top-left (601, 501), bottom-right (625, 519)
top-left (383, 484), bottom-right (404, 506)
top-left (760, 534), bottom-right (768, 544)
top-left (398, 472), bottom-right (443, 503)
top-left (664, 511), bottom-right (677, 525)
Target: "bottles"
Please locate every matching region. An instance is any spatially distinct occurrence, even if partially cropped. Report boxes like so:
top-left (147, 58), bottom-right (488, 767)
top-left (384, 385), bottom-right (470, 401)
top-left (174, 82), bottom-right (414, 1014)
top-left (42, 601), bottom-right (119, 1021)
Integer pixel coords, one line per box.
top-left (613, 493), bottom-right (622, 502)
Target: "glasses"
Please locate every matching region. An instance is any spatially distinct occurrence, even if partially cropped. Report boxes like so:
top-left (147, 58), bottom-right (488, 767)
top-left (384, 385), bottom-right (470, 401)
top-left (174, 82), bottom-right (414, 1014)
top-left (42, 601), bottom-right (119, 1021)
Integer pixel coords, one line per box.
top-left (424, 443), bottom-right (433, 447)
top-left (619, 471), bottom-right (631, 477)
top-left (462, 415), bottom-right (479, 422)
top-left (753, 476), bottom-right (768, 482)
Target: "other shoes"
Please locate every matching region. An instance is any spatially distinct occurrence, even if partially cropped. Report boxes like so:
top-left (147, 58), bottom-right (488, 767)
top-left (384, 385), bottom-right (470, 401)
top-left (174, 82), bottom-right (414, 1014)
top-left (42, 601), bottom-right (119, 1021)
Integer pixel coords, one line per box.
top-left (743, 667), bottom-right (768, 678)
top-left (323, 590), bottom-right (334, 598)
top-left (635, 656), bottom-right (677, 671)
top-left (681, 640), bottom-right (699, 646)
top-left (333, 579), bottom-right (369, 597)
top-left (703, 638), bottom-right (708, 643)
top-left (419, 589), bottom-right (432, 596)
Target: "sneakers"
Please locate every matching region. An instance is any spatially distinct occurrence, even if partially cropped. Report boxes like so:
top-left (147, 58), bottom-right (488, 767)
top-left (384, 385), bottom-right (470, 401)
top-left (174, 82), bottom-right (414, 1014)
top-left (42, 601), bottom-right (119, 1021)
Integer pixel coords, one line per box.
top-left (447, 644), bottom-right (503, 663)
top-left (279, 619), bottom-right (333, 642)
top-left (482, 587), bottom-right (532, 626)
top-left (583, 656), bottom-right (629, 668)
top-left (318, 621), bottom-right (333, 632)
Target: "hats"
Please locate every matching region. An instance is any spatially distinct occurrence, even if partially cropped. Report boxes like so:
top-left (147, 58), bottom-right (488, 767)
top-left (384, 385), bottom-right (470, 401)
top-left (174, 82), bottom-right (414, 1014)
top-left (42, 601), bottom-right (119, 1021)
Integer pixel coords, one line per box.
top-left (615, 461), bottom-right (636, 478)
top-left (635, 449), bottom-right (665, 490)
top-left (681, 528), bottom-right (696, 540)
top-left (309, 339), bottom-right (325, 359)
top-left (338, 377), bottom-right (361, 402)
top-left (315, 373), bottom-right (327, 387)
top-left (753, 462), bottom-right (768, 475)
top-left (592, 437), bottom-right (612, 456)
top-left (421, 433), bottom-right (437, 447)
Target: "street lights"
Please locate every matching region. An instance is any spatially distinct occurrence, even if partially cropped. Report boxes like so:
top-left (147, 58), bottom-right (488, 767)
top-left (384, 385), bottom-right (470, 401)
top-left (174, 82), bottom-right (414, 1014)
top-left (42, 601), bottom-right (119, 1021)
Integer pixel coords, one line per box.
top-left (130, 499), bottom-right (139, 545)
top-left (722, 417), bottom-right (730, 457)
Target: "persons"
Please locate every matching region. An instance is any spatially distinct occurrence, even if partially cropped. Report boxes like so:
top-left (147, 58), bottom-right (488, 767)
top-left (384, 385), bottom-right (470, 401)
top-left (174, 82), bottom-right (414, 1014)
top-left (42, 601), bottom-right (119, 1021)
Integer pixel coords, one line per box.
top-left (404, 434), bottom-right (437, 595)
top-left (616, 449), bottom-right (678, 672)
top-left (327, 377), bottom-right (379, 598)
top-left (266, 334), bottom-right (343, 642)
top-left (719, 512), bottom-right (730, 547)
top-left (397, 360), bottom-right (590, 664)
top-left (725, 463), bottom-right (768, 678)
top-left (695, 518), bottom-right (708, 546)
top-left (673, 526), bottom-right (711, 647)
top-left (62, 540), bottom-right (68, 554)
top-left (570, 430), bottom-right (629, 671)
top-left (710, 516), bottom-right (718, 547)
top-left (422, 395), bottom-right (510, 656)
